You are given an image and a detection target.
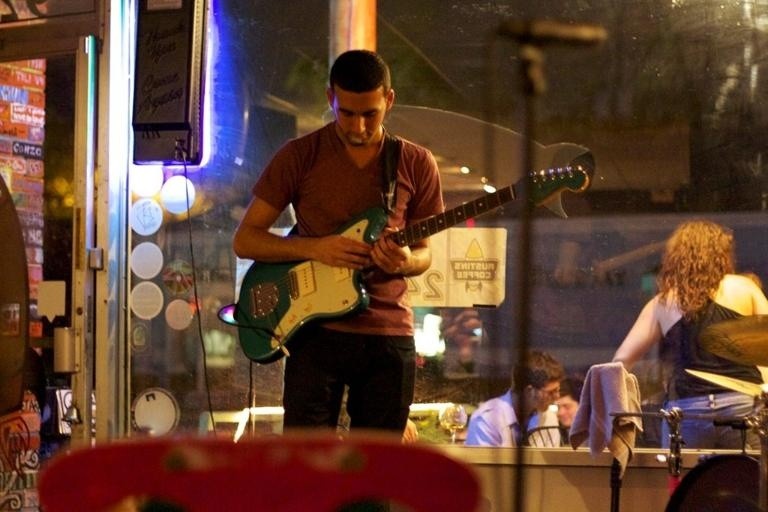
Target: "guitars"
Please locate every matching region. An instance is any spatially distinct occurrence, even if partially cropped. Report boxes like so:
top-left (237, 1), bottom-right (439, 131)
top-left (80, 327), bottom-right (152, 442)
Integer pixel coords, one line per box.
top-left (238, 165), bottom-right (589, 365)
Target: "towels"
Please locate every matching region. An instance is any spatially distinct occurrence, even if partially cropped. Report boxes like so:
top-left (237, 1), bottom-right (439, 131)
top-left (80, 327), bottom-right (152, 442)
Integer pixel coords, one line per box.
top-left (569, 360), bottom-right (644, 482)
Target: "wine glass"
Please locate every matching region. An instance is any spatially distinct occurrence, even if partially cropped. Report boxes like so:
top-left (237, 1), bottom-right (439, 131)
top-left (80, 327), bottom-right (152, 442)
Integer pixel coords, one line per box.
top-left (436, 402), bottom-right (468, 443)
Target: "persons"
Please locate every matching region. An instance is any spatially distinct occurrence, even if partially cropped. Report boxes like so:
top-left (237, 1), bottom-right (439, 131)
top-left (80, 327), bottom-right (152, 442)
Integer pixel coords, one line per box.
top-left (612, 220), bottom-right (768, 449)
top-left (555, 376), bottom-right (585, 428)
top-left (233, 49), bottom-right (444, 437)
top-left (464, 348), bottom-right (562, 449)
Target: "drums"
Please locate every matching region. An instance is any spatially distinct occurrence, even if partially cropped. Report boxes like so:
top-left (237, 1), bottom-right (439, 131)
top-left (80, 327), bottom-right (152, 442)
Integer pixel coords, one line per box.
top-left (665, 452), bottom-right (768, 512)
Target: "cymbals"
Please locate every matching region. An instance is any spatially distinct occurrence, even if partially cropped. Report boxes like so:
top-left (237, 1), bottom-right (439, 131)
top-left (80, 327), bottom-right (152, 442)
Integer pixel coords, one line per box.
top-left (698, 314), bottom-right (767, 366)
top-left (685, 368), bottom-right (767, 397)
